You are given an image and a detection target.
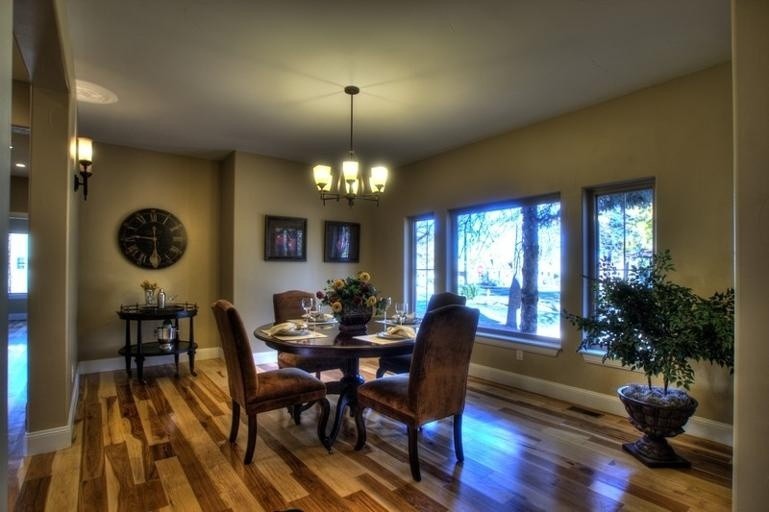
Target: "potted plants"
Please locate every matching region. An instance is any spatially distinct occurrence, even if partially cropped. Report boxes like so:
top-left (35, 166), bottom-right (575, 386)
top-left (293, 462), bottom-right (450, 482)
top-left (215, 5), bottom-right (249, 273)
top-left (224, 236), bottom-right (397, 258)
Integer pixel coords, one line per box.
top-left (561, 251), bottom-right (733, 467)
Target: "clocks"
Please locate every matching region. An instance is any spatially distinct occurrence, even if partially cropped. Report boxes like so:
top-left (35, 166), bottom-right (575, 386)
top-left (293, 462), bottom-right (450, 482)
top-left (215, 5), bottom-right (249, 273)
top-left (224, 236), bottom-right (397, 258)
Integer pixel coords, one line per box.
top-left (118, 207), bottom-right (187, 270)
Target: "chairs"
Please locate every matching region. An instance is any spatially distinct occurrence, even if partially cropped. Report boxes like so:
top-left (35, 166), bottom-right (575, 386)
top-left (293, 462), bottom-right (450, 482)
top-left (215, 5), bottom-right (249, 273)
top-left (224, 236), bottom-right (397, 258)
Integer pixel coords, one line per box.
top-left (376, 293), bottom-right (466, 378)
top-left (353, 306), bottom-right (480, 481)
top-left (212, 300), bottom-right (331, 465)
top-left (273, 290), bottom-right (347, 418)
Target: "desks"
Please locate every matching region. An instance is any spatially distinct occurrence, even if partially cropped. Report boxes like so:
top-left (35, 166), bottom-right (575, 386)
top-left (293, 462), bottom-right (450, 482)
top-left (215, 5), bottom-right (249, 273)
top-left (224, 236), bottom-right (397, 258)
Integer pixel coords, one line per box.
top-left (118, 301), bottom-right (198, 383)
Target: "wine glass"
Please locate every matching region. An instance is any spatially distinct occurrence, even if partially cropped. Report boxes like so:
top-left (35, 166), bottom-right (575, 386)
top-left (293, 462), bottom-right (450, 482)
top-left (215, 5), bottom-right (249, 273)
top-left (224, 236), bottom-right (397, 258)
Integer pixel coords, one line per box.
top-left (302, 297), bottom-right (328, 324)
top-left (383, 297), bottom-right (419, 327)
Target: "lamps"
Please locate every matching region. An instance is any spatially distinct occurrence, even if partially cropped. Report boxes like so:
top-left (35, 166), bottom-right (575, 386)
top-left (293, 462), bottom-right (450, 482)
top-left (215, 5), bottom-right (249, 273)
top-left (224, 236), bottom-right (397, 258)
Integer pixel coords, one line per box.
top-left (313, 87), bottom-right (389, 205)
top-left (74, 136), bottom-right (94, 197)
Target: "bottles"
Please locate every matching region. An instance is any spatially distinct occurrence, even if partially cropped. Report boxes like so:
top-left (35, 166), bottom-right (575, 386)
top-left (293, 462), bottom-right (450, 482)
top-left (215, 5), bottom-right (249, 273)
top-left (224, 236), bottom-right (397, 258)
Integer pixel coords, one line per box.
top-left (157, 288), bottom-right (166, 309)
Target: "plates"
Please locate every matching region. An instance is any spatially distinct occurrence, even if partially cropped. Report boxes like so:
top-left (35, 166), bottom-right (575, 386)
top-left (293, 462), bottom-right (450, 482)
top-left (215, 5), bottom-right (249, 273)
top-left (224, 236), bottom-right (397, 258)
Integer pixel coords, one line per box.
top-left (376, 331), bottom-right (409, 339)
top-left (301, 314), bottom-right (333, 321)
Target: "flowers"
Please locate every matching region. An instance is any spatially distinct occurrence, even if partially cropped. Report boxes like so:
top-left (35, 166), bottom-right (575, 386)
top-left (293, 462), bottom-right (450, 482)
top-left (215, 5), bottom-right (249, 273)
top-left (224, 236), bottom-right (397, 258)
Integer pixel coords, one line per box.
top-left (316, 271), bottom-right (387, 313)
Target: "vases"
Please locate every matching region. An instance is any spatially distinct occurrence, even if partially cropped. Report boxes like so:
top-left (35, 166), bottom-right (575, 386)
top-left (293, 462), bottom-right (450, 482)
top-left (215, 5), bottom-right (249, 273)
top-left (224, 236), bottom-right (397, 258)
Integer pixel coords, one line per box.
top-left (334, 306), bottom-right (373, 332)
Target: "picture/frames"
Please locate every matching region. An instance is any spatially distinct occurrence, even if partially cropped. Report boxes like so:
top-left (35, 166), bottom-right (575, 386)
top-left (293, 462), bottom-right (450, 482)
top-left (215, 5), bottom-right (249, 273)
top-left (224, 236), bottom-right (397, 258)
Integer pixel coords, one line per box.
top-left (265, 214), bottom-right (307, 261)
top-left (324, 220), bottom-right (360, 263)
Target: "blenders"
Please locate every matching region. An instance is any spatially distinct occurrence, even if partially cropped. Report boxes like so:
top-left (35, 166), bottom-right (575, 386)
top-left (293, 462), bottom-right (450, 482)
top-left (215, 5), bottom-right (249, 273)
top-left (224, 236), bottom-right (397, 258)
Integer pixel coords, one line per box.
top-left (153, 320), bottom-right (177, 353)
